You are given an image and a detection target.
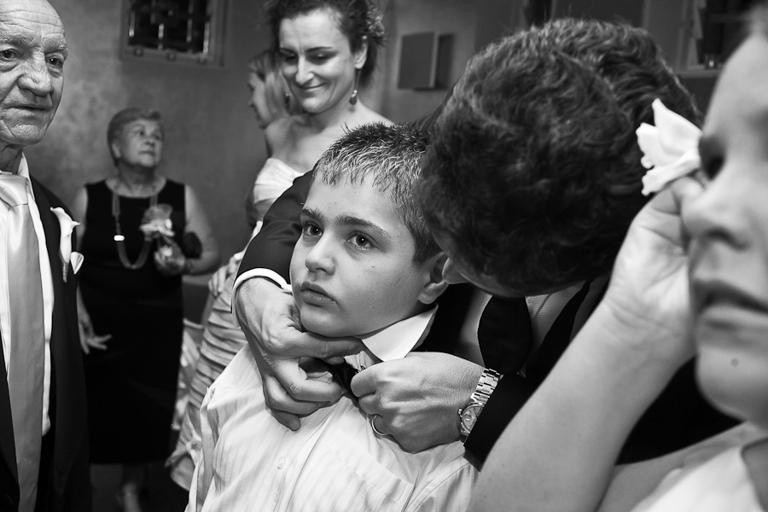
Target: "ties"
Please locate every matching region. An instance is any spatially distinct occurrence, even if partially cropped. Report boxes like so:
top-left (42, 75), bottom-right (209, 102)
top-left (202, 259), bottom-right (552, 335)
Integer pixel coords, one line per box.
top-left (476, 293), bottom-right (535, 375)
top-left (0, 175), bottom-right (45, 511)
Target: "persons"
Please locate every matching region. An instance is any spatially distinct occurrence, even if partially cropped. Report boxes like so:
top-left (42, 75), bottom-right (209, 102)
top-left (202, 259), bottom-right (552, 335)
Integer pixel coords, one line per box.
top-left (164, 1), bottom-right (398, 512)
top-left (466, 2), bottom-right (768, 512)
top-left (69, 108), bottom-right (221, 512)
top-left (232, 19), bottom-right (745, 472)
top-left (0, 0), bottom-right (84, 512)
top-left (187, 121), bottom-right (479, 511)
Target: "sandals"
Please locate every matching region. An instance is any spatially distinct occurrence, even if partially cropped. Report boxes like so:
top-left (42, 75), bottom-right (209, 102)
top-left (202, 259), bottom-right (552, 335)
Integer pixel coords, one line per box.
top-left (116, 464), bottom-right (156, 512)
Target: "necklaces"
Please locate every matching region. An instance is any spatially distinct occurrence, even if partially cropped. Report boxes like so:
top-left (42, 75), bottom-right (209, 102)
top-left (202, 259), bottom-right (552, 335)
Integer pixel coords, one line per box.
top-left (112, 177), bottom-right (158, 269)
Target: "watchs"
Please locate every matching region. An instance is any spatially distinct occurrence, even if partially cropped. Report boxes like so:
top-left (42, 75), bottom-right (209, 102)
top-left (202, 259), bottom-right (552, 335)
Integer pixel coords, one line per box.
top-left (458, 368), bottom-right (503, 440)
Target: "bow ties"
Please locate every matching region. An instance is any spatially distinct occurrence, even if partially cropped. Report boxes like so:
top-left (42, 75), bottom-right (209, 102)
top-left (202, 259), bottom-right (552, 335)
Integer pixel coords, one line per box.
top-left (298, 357), bottom-right (359, 396)
